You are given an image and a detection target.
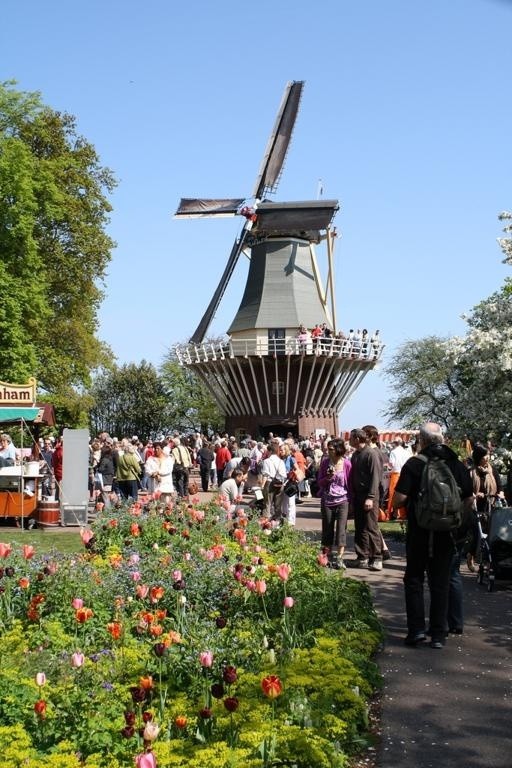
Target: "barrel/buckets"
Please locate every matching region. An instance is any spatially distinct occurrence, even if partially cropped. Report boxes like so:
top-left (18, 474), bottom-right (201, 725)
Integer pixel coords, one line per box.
top-left (63, 502), bottom-right (88, 527)
top-left (36, 500), bottom-right (60, 526)
top-left (25, 460), bottom-right (40, 476)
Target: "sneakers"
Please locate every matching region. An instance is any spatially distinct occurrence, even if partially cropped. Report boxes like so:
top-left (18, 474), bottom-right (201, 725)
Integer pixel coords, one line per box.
top-left (383, 550), bottom-right (391, 560)
top-left (335, 557), bottom-right (347, 569)
top-left (466, 555), bottom-right (476, 573)
top-left (371, 561), bottom-right (382, 570)
top-left (351, 558), bottom-right (369, 568)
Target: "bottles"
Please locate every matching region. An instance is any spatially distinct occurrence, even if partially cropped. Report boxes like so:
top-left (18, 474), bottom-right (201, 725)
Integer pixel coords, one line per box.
top-left (36, 476), bottom-right (50, 502)
top-left (495, 497), bottom-right (502, 507)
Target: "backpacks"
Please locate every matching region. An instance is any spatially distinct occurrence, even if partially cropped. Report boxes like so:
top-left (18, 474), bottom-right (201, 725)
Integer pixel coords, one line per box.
top-left (268, 459), bottom-right (284, 493)
top-left (413, 452), bottom-right (462, 531)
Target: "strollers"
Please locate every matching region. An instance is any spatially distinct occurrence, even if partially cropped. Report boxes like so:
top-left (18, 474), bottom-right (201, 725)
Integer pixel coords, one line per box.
top-left (474, 495), bottom-right (512, 592)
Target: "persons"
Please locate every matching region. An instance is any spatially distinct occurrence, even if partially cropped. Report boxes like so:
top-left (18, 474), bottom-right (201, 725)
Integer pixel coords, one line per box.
top-left (390, 423), bottom-right (511, 649)
top-left (296, 321), bottom-right (382, 362)
top-left (1, 425), bottom-right (422, 572)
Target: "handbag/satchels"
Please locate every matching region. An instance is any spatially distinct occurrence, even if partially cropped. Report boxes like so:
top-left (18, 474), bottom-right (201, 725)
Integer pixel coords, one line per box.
top-left (189, 483), bottom-right (198, 495)
top-left (285, 480), bottom-right (298, 496)
top-left (174, 462), bottom-right (185, 473)
